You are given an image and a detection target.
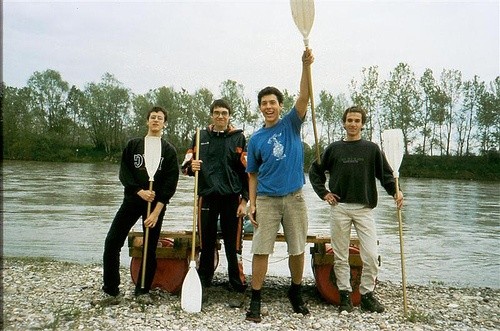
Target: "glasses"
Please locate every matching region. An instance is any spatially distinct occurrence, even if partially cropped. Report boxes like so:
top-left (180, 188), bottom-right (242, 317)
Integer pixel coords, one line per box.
top-left (213, 111), bottom-right (229, 116)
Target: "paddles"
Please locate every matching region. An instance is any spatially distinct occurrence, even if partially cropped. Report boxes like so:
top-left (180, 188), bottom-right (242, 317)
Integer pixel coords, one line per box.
top-left (383, 128), bottom-right (407, 318)
top-left (289, 0), bottom-right (320, 164)
top-left (141, 135), bottom-right (162, 290)
top-left (181, 126), bottom-right (203, 313)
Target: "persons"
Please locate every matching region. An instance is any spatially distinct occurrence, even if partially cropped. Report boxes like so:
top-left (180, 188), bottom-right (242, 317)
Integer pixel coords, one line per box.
top-left (245, 49), bottom-right (315, 322)
top-left (92, 107), bottom-right (179, 306)
top-left (309, 107), bottom-right (403, 313)
top-left (179, 99), bottom-right (250, 290)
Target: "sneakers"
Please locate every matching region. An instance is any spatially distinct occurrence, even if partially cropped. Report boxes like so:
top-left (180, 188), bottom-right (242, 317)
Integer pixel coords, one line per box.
top-left (287, 289), bottom-right (311, 316)
top-left (229, 290), bottom-right (245, 307)
top-left (338, 291), bottom-right (354, 313)
top-left (361, 292), bottom-right (385, 313)
top-left (245, 301), bottom-right (261, 322)
top-left (137, 294), bottom-right (153, 305)
top-left (90, 292), bottom-right (120, 307)
top-left (202, 286), bottom-right (208, 303)
